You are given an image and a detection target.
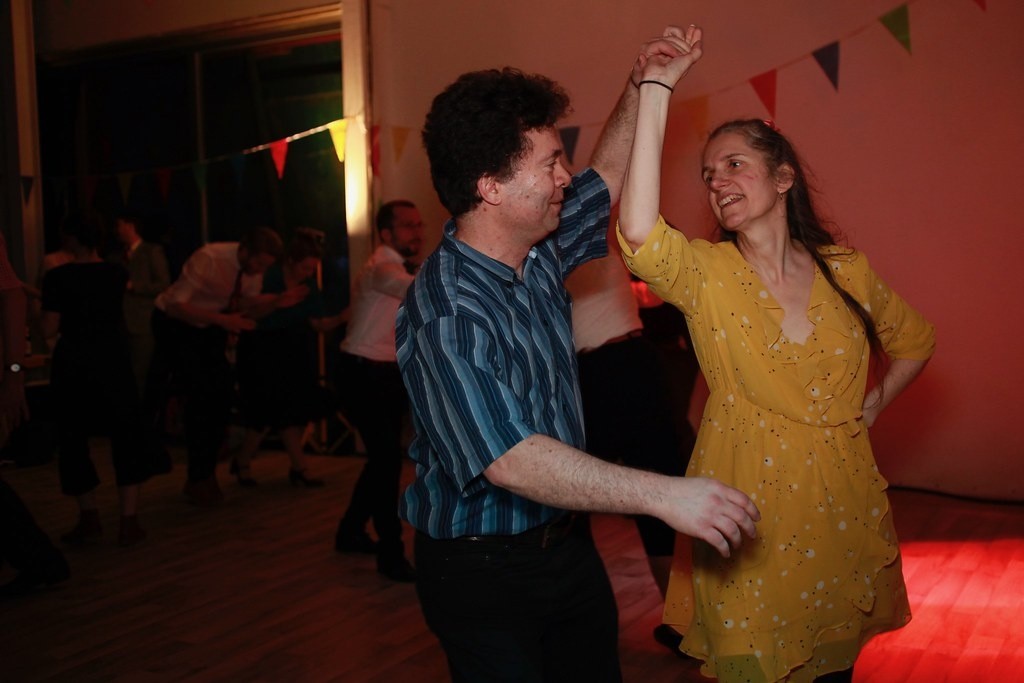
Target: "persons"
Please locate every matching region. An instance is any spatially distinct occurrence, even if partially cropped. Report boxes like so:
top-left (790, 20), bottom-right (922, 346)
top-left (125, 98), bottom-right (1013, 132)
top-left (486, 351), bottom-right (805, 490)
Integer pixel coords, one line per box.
top-left (564, 245), bottom-right (691, 662)
top-left (339, 200), bottom-right (426, 583)
top-left (1, 209), bottom-right (352, 607)
top-left (619, 24), bottom-right (939, 683)
top-left (384, 34), bottom-right (762, 683)
top-left (630, 220), bottom-right (695, 476)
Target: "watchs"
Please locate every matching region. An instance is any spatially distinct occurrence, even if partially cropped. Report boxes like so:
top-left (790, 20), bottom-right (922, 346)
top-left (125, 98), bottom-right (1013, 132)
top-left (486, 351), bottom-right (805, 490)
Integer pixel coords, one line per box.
top-left (3, 362), bottom-right (28, 373)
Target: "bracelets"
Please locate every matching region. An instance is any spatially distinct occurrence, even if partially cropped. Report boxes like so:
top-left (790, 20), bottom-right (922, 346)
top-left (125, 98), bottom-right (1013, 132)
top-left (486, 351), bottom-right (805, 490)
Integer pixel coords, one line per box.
top-left (638, 80), bottom-right (674, 92)
top-left (630, 75), bottom-right (639, 90)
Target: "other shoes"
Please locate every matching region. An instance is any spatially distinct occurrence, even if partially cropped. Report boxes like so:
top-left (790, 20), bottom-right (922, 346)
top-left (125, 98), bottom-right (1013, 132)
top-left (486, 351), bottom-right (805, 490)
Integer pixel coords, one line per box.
top-left (0, 570), bottom-right (72, 597)
top-left (653, 622), bottom-right (689, 659)
top-left (229, 459), bottom-right (256, 486)
top-left (185, 479), bottom-right (225, 504)
top-left (59, 508), bottom-right (102, 543)
top-left (288, 467), bottom-right (324, 488)
top-left (377, 557), bottom-right (416, 583)
top-left (335, 535), bottom-right (374, 554)
top-left (118, 513), bottom-right (144, 546)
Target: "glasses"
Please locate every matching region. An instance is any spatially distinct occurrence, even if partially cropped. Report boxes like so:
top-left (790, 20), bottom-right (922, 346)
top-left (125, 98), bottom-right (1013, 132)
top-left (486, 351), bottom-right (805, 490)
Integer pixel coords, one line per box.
top-left (395, 222), bottom-right (427, 230)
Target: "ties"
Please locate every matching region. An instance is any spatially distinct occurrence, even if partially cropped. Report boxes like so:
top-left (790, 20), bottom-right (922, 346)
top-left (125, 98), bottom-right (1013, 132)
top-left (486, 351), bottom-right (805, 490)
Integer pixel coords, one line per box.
top-left (230, 271), bottom-right (243, 302)
top-left (403, 260), bottom-right (415, 275)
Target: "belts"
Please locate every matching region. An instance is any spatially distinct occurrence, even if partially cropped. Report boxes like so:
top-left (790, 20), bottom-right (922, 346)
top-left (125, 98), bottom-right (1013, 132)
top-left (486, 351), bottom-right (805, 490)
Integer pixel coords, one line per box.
top-left (464, 515), bottom-right (576, 549)
